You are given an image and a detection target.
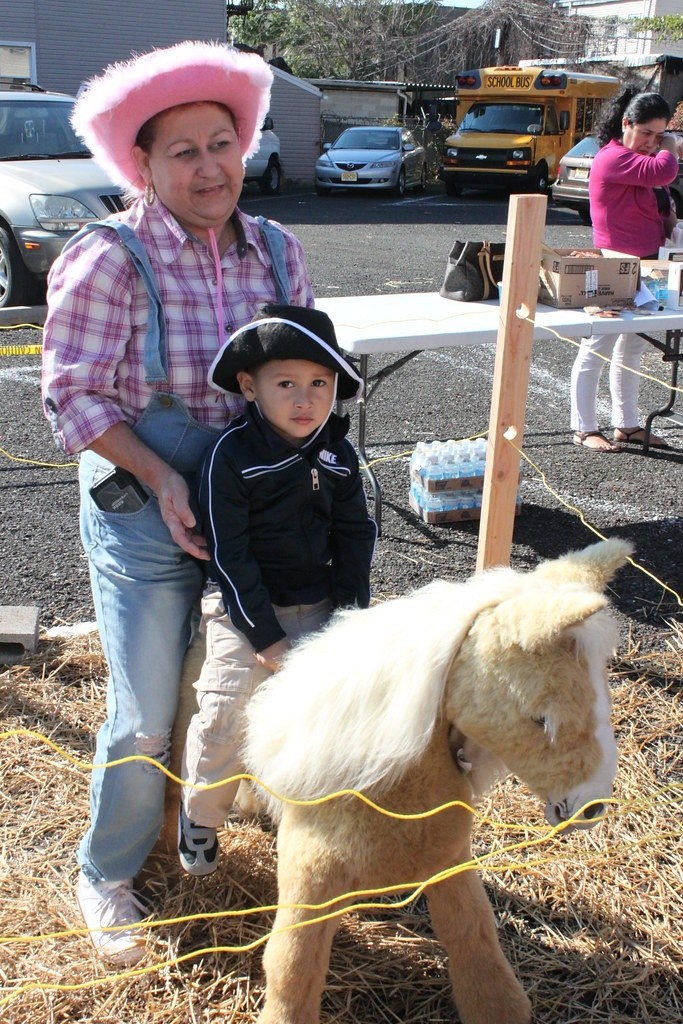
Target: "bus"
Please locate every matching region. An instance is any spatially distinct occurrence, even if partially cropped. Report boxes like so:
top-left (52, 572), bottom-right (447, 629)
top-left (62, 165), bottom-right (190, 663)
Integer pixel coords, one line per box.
top-left (432, 65), bottom-right (621, 199)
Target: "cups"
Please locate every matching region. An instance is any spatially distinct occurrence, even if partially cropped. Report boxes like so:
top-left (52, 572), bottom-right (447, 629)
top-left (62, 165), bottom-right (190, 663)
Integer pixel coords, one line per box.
top-left (674, 222), bottom-right (683, 247)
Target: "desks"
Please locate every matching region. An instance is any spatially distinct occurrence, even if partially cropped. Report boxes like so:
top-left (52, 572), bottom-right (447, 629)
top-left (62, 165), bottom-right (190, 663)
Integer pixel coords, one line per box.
top-left (310, 290), bottom-right (683, 539)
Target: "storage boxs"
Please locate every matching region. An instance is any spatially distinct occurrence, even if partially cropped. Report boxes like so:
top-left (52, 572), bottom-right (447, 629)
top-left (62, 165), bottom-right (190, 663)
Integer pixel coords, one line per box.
top-left (408, 443), bottom-right (524, 522)
top-left (538, 244), bottom-right (641, 309)
top-left (640, 245), bottom-right (683, 312)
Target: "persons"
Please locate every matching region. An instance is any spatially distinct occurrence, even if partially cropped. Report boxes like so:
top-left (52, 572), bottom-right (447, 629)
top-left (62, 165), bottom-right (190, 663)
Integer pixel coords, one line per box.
top-left (41, 39), bottom-right (313, 967)
top-left (177, 304), bottom-right (382, 876)
top-left (569, 81), bottom-right (679, 453)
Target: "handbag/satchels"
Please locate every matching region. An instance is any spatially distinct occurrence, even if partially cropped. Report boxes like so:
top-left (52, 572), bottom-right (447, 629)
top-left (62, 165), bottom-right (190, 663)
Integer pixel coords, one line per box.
top-left (439, 239), bottom-right (507, 302)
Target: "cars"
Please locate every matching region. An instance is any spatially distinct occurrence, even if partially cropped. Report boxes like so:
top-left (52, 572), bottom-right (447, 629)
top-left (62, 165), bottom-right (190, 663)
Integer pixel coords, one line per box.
top-left (243, 116), bottom-right (284, 194)
top-left (313, 126), bottom-right (428, 198)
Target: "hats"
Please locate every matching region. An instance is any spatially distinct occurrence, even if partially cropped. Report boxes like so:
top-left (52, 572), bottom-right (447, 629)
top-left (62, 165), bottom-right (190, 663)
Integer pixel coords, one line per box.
top-left (208, 305), bottom-right (365, 403)
top-left (71, 39), bottom-right (274, 199)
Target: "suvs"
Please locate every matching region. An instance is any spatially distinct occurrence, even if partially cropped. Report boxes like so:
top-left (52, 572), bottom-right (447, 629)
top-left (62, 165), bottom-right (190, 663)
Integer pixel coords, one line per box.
top-left (549, 131), bottom-right (683, 223)
top-left (0, 80), bottom-right (135, 309)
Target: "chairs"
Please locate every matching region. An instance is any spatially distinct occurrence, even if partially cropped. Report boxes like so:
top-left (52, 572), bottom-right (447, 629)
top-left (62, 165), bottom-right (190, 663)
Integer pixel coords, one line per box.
top-left (352, 134), bottom-right (368, 149)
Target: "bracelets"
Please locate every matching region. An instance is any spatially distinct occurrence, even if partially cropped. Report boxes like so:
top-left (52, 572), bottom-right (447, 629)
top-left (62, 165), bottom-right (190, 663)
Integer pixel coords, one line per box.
top-left (662, 132), bottom-right (678, 141)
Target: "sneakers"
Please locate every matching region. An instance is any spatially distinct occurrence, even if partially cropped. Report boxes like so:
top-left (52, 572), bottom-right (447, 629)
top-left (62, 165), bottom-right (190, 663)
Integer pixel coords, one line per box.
top-left (74, 869), bottom-right (156, 966)
top-left (176, 798), bottom-right (220, 875)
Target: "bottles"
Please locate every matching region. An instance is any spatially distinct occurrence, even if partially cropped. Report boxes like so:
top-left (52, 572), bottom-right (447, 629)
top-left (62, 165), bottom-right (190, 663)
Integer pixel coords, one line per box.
top-left (408, 437), bottom-right (523, 512)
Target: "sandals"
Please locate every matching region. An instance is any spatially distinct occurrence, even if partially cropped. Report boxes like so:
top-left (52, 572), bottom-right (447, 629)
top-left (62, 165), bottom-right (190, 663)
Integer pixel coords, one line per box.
top-left (613, 426), bottom-right (668, 448)
top-left (572, 430), bottom-right (620, 452)
